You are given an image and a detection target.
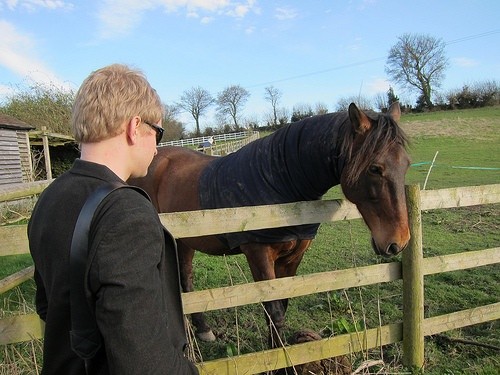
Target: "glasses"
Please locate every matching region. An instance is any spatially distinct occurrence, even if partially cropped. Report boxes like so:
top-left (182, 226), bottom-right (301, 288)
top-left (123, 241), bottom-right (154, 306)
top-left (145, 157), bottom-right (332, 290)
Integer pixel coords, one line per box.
top-left (143, 120), bottom-right (165, 145)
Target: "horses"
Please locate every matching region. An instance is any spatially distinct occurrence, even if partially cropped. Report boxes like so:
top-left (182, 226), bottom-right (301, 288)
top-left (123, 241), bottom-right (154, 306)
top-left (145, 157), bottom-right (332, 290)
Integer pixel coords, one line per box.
top-left (128, 100), bottom-right (411, 349)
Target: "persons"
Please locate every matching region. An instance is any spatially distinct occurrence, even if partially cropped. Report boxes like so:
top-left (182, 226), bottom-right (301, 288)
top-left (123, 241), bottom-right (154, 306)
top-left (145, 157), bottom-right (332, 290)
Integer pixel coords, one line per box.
top-left (26, 63), bottom-right (201, 375)
